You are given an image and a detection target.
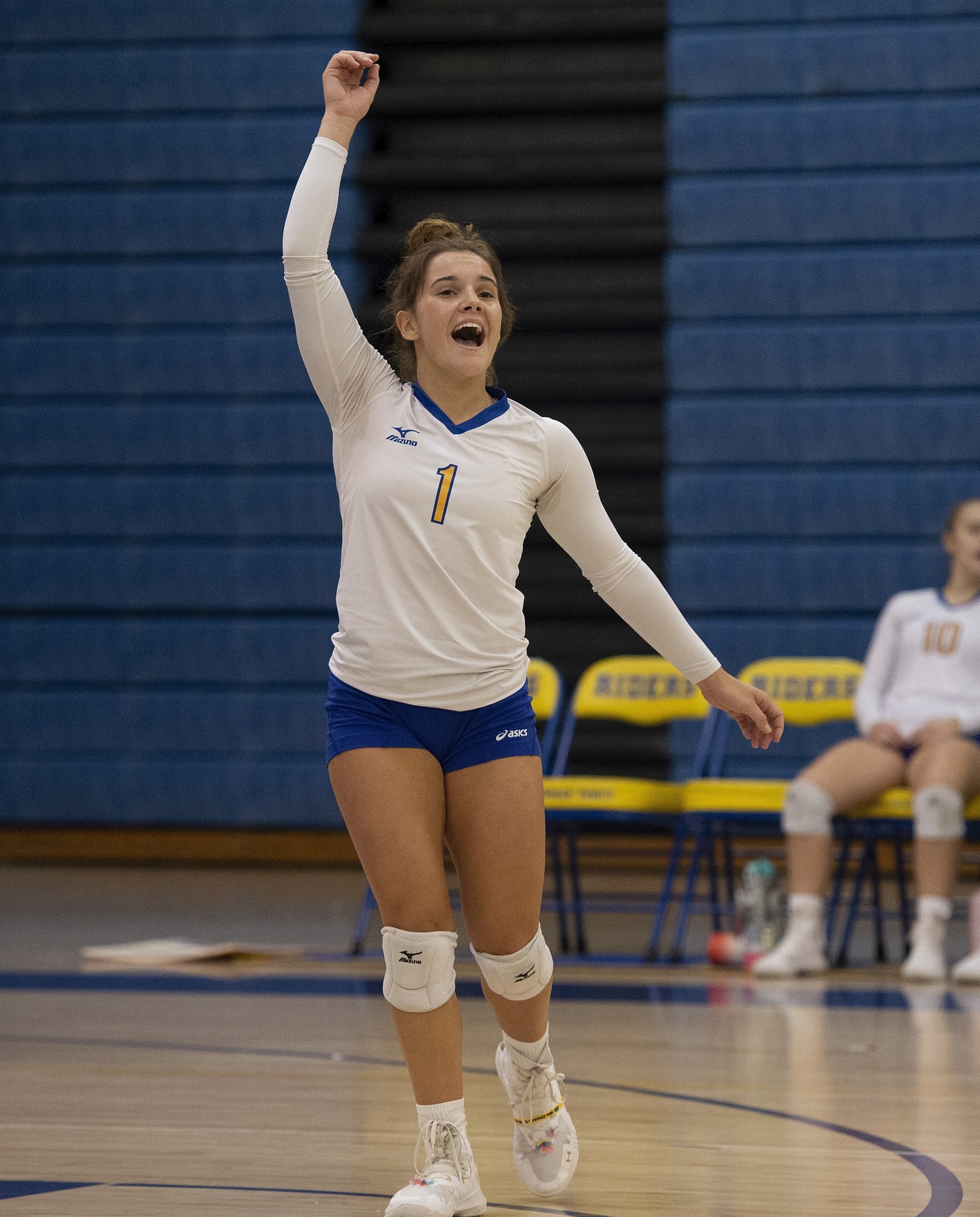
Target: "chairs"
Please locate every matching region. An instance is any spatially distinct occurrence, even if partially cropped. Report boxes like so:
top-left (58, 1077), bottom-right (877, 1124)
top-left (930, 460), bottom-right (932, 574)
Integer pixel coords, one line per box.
top-left (354, 659), bottom-right (980, 962)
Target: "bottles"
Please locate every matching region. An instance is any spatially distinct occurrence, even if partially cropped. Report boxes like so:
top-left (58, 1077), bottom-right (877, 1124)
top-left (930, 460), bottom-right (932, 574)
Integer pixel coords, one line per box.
top-left (734, 860), bottom-right (779, 971)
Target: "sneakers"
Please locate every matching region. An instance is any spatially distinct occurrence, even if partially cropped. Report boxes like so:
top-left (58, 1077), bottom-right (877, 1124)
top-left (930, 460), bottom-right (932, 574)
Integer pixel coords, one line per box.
top-left (495, 1036), bottom-right (579, 1198)
top-left (753, 909), bottom-right (829, 977)
top-left (384, 1105), bottom-right (488, 1217)
top-left (900, 918), bottom-right (947, 981)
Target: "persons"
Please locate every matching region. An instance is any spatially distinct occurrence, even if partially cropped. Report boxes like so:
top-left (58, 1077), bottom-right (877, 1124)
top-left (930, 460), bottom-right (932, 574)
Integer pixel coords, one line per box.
top-left (951, 887), bottom-right (980, 983)
top-left (752, 497), bottom-right (980, 983)
top-left (280, 49), bottom-right (784, 1217)
